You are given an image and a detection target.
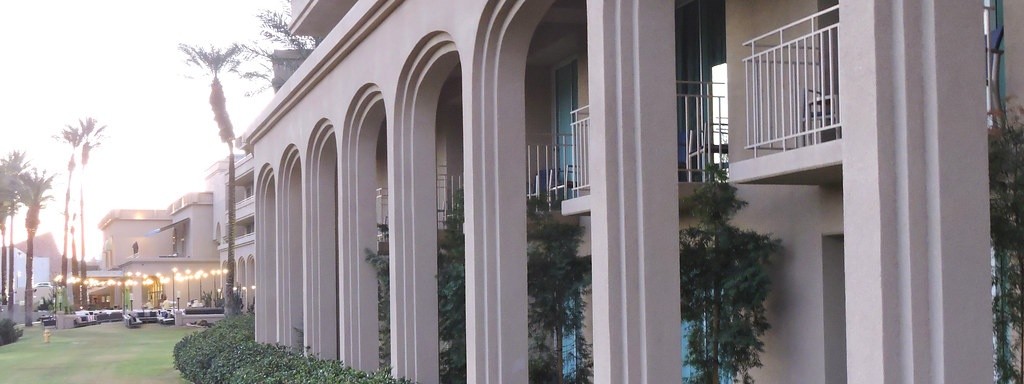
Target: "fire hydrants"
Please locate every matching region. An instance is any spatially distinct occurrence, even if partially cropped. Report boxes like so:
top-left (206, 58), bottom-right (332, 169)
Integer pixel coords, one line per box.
top-left (43, 329), bottom-right (50, 342)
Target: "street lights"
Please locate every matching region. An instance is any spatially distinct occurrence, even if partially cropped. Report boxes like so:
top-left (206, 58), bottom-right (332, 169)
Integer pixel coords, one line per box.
top-left (171, 268), bottom-right (176, 308)
top-left (184, 269), bottom-right (193, 294)
top-left (195, 271), bottom-right (207, 303)
top-left (211, 270), bottom-right (220, 300)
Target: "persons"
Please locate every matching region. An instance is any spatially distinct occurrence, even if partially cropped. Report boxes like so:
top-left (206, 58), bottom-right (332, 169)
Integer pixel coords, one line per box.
top-left (161, 297), bottom-right (169, 311)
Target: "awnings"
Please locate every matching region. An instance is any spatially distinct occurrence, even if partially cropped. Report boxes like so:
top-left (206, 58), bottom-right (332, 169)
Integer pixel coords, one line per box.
top-left (145, 217), bottom-right (189, 237)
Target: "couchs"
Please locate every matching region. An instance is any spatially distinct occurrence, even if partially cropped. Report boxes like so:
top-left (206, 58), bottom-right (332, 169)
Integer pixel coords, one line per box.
top-left (75, 311), bottom-right (174, 327)
